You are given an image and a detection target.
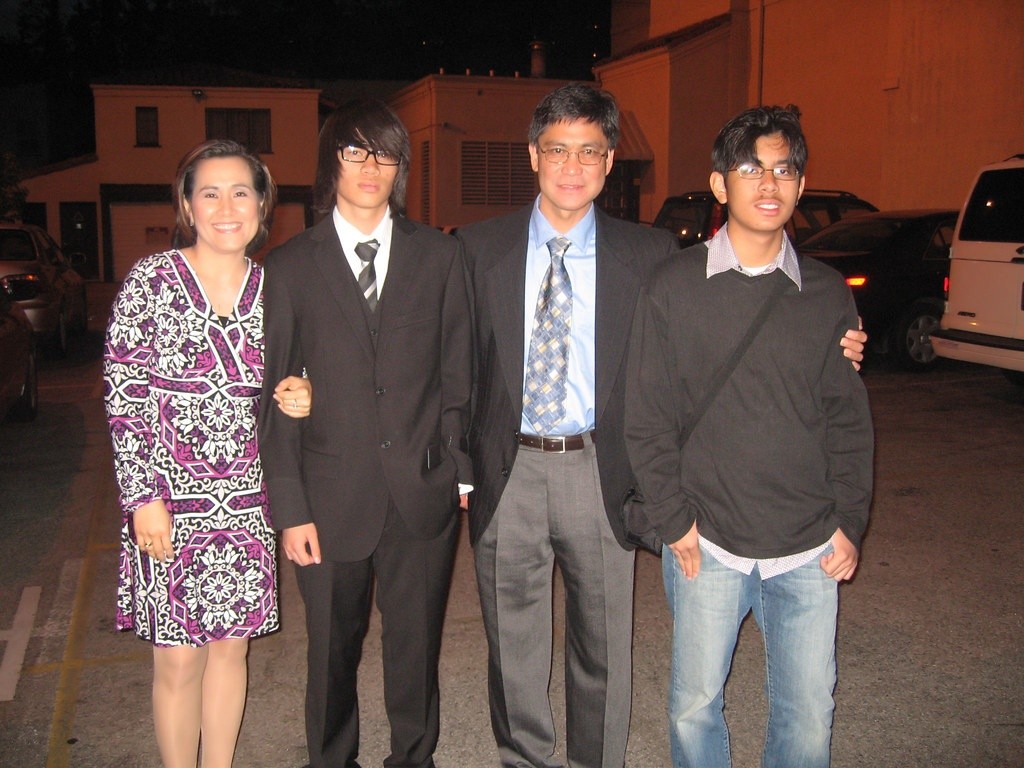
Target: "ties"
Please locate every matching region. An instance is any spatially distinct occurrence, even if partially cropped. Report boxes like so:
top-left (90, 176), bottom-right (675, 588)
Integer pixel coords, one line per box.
top-left (522, 238), bottom-right (574, 437)
top-left (354, 238), bottom-right (380, 314)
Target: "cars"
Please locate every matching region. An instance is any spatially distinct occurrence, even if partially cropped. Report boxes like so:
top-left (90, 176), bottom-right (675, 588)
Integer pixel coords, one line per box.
top-left (794, 196), bottom-right (964, 373)
top-left (2, 214), bottom-right (92, 356)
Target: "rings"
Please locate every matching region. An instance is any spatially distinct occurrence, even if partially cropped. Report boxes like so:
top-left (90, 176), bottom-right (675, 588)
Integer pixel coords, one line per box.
top-left (145, 542), bottom-right (153, 548)
top-left (294, 400), bottom-right (297, 407)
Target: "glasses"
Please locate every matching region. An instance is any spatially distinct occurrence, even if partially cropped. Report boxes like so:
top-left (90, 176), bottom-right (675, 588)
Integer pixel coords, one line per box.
top-left (335, 145), bottom-right (401, 165)
top-left (535, 140), bottom-right (610, 165)
top-left (725, 163), bottom-right (801, 181)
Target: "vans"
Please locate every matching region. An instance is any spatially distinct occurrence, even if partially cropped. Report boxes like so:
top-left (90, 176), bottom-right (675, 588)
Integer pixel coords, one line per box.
top-left (929, 148), bottom-right (1023, 389)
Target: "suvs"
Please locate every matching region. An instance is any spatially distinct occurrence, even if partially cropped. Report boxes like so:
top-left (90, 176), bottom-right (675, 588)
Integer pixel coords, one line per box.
top-left (648, 185), bottom-right (880, 273)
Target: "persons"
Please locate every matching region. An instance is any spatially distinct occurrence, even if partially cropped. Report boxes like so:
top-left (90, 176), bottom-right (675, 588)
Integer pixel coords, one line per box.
top-left (102, 139), bottom-right (312, 767)
top-left (257, 94), bottom-right (473, 768)
top-left (456, 79), bottom-right (867, 767)
top-left (623, 107), bottom-right (876, 768)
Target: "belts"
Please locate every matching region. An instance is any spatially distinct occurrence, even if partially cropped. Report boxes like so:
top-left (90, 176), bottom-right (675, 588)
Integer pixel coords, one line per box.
top-left (519, 428), bottom-right (597, 455)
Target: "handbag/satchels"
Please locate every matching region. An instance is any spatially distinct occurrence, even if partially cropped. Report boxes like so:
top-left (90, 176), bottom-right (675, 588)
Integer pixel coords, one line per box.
top-left (621, 481), bottom-right (665, 556)
